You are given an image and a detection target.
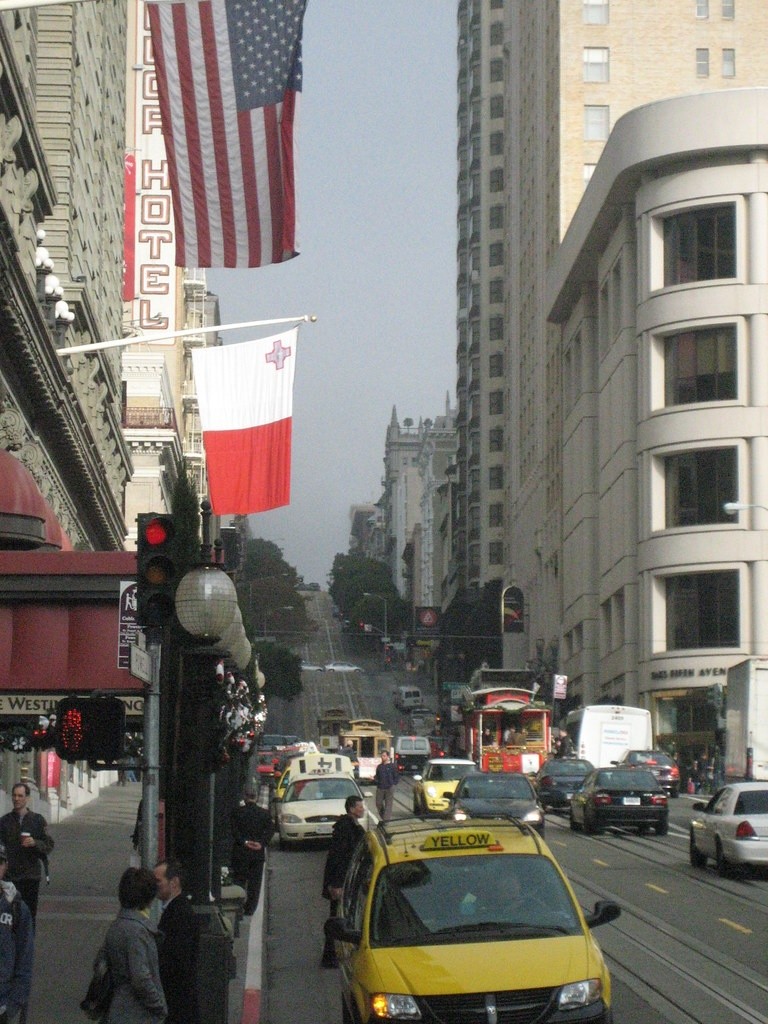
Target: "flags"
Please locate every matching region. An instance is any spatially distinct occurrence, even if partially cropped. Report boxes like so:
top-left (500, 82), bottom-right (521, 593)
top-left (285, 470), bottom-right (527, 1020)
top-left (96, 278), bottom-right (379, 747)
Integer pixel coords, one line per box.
top-left (191, 325), bottom-right (300, 515)
top-left (150, 0), bottom-right (305, 272)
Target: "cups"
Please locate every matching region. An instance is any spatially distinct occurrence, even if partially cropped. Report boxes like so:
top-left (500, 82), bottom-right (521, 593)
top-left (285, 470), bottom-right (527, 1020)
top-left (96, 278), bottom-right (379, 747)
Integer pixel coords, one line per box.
top-left (20, 831), bottom-right (31, 847)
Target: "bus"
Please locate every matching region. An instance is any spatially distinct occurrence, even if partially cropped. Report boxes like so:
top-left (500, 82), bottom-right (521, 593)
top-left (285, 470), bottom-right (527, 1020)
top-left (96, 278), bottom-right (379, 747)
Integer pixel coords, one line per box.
top-left (464, 686), bottom-right (552, 773)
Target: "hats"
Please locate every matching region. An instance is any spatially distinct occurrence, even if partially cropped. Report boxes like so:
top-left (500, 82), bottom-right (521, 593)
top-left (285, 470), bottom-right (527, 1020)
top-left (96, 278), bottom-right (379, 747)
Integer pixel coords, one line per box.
top-left (0, 845), bottom-right (8, 858)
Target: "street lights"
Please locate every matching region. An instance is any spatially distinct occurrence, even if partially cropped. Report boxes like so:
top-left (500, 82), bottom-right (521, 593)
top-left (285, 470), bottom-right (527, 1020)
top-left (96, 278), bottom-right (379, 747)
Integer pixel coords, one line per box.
top-left (363, 591), bottom-right (388, 664)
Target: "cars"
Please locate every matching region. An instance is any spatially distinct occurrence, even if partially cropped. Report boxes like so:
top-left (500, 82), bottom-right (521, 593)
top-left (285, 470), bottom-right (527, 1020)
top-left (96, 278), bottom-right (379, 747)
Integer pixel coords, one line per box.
top-left (610, 749), bottom-right (681, 799)
top-left (324, 661), bottom-right (363, 673)
top-left (412, 758), bottom-right (482, 818)
top-left (566, 767), bottom-right (669, 836)
top-left (442, 771), bottom-right (551, 840)
top-left (688, 782), bottom-right (768, 879)
top-left (272, 770), bottom-right (375, 851)
top-left (325, 809), bottom-right (622, 1024)
top-left (256, 707), bottom-right (447, 811)
top-left (301, 661), bottom-right (323, 673)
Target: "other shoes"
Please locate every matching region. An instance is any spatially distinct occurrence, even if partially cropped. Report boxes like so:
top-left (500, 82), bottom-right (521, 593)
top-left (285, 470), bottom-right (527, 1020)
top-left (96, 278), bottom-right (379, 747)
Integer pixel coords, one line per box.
top-left (322, 959), bottom-right (340, 968)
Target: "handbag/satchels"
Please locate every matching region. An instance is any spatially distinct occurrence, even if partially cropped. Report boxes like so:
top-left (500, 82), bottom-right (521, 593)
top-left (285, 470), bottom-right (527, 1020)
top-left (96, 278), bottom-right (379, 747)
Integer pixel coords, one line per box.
top-left (80, 960), bottom-right (112, 1021)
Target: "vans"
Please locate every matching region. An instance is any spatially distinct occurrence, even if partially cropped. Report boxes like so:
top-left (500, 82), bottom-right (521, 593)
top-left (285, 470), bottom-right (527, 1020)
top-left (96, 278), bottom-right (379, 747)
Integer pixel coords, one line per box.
top-left (566, 705), bottom-right (652, 768)
top-left (392, 686), bottom-right (422, 712)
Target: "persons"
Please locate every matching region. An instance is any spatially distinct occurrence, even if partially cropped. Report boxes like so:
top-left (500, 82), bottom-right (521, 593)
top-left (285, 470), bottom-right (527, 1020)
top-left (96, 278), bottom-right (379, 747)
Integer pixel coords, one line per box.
top-left (375, 750), bottom-right (400, 822)
top-left (555, 726), bottom-right (575, 759)
top-left (483, 729), bottom-right (493, 746)
top-left (98, 866), bottom-right (169, 1024)
top-left (338, 740), bottom-right (359, 766)
top-left (319, 795), bottom-right (367, 969)
top-left (0, 845), bottom-right (35, 1024)
top-left (0, 783), bottom-right (55, 937)
top-left (152, 859), bottom-right (201, 1024)
top-left (228, 788), bottom-right (275, 916)
top-left (503, 725), bottom-right (516, 747)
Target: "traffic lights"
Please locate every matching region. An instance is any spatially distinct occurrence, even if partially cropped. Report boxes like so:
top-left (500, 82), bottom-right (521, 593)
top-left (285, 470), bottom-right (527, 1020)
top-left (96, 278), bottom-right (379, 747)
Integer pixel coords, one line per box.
top-left (134, 511), bottom-right (178, 626)
top-left (57, 697), bottom-right (124, 763)
